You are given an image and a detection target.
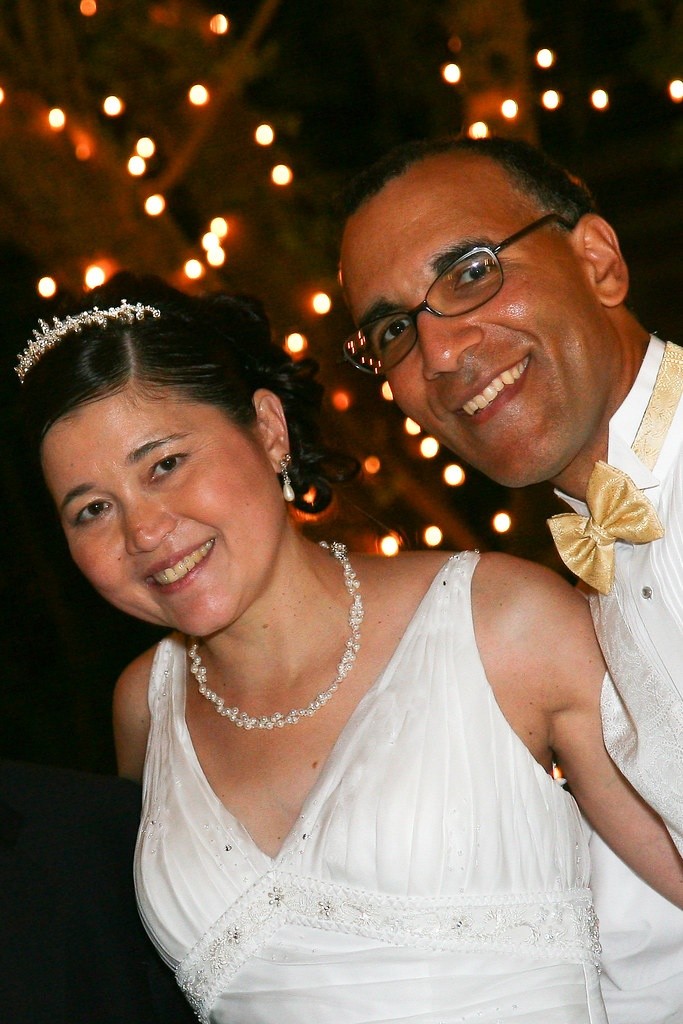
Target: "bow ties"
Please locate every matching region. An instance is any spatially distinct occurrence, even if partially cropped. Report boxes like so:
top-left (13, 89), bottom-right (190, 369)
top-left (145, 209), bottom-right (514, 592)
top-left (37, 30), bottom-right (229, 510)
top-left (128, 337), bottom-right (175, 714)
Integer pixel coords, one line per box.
top-left (546, 341), bottom-right (683, 595)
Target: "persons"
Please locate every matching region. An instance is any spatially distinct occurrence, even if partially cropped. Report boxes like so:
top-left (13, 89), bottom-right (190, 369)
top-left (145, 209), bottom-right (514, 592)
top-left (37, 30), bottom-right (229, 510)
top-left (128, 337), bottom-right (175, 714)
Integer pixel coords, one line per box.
top-left (14, 272), bottom-right (683, 1024)
top-left (340, 137), bottom-right (683, 868)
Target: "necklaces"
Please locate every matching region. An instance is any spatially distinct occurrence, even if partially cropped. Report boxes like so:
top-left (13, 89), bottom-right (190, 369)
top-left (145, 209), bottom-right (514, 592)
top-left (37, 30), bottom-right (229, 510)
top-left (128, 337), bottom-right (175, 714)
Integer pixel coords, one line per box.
top-left (186, 537), bottom-right (362, 732)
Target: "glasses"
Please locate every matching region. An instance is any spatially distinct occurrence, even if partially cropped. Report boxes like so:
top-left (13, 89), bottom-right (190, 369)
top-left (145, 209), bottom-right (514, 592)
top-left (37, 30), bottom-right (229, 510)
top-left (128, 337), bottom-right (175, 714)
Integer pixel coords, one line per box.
top-left (342, 212), bottom-right (575, 375)
